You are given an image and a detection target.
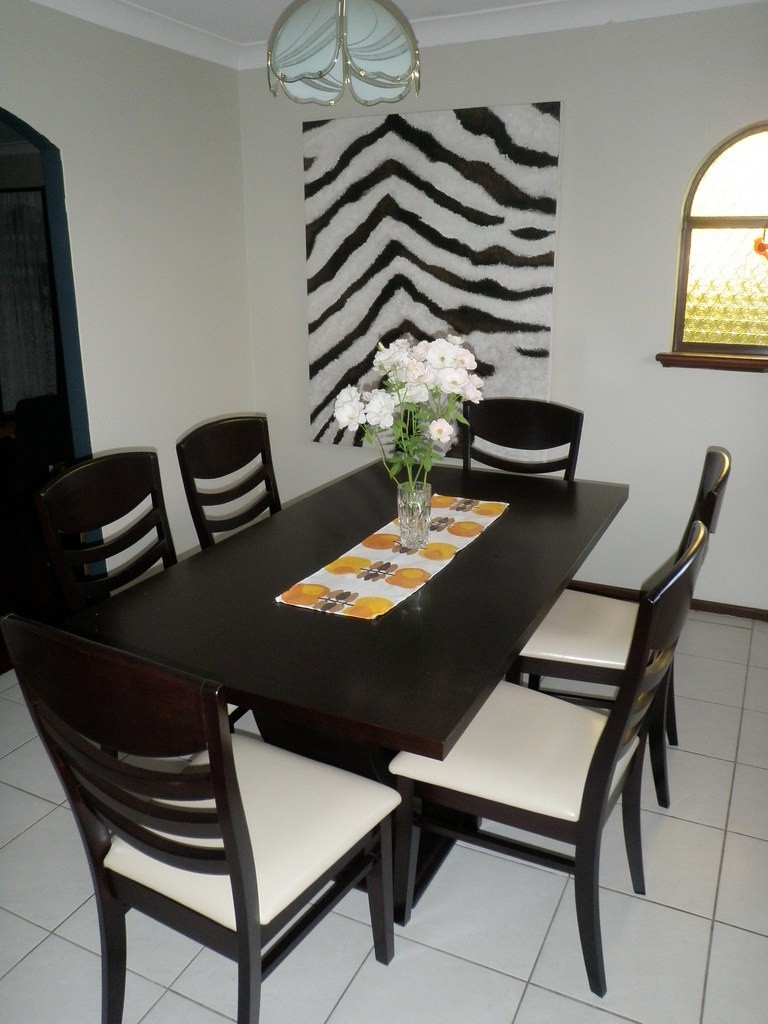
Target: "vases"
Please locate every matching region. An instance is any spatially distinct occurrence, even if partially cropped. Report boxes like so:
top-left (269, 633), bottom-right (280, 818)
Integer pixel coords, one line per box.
top-left (395, 481), bottom-right (432, 552)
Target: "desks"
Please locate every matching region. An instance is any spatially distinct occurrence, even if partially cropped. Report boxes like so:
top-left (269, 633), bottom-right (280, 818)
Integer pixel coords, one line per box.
top-left (59, 460), bottom-right (630, 911)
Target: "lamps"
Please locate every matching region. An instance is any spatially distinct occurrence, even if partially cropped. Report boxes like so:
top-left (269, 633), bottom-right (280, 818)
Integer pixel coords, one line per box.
top-left (264, 0), bottom-right (423, 107)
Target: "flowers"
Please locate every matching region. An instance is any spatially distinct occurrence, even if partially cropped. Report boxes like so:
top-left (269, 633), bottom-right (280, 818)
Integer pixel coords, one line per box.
top-left (332, 334), bottom-right (484, 493)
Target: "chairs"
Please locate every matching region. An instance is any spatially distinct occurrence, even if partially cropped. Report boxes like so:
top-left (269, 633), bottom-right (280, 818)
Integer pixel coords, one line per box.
top-left (175, 415), bottom-right (283, 553)
top-left (385, 517), bottom-right (710, 1002)
top-left (458, 396), bottom-right (584, 480)
top-left (37, 446), bottom-right (178, 616)
top-left (0, 614), bottom-right (398, 1024)
top-left (501, 443), bottom-right (733, 808)
